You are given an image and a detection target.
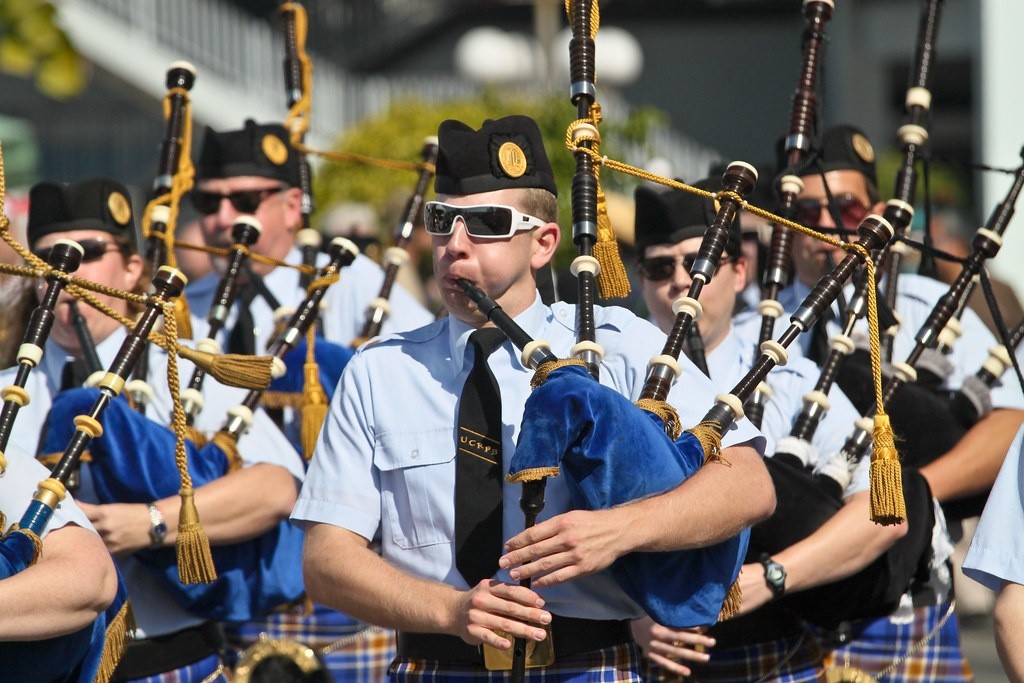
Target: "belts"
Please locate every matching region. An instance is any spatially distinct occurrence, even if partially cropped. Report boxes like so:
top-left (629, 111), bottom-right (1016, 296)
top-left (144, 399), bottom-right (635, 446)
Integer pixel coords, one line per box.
top-left (397, 617), bottom-right (634, 672)
top-left (108, 619), bottom-right (226, 683)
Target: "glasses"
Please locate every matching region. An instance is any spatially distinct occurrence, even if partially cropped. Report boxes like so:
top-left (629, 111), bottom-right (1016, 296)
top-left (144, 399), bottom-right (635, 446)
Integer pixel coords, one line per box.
top-left (32, 238), bottom-right (132, 264)
top-left (636, 252), bottom-right (736, 280)
top-left (792, 192), bottom-right (873, 230)
top-left (424, 201), bottom-right (547, 238)
top-left (194, 185), bottom-right (291, 215)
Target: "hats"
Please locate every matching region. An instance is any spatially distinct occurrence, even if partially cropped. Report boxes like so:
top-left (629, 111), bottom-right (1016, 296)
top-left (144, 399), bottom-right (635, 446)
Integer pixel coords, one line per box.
top-left (634, 175), bottom-right (741, 250)
top-left (196, 119), bottom-right (312, 197)
top-left (27, 177), bottom-right (139, 255)
top-left (434, 114), bottom-right (558, 199)
top-left (776, 125), bottom-right (877, 191)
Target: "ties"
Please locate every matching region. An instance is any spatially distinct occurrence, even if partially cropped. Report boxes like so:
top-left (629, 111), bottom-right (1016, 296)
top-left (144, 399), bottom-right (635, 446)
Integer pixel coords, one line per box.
top-left (455, 327), bottom-right (506, 589)
top-left (228, 282), bottom-right (259, 354)
top-left (59, 357), bottom-right (90, 391)
top-left (807, 306), bottom-right (835, 366)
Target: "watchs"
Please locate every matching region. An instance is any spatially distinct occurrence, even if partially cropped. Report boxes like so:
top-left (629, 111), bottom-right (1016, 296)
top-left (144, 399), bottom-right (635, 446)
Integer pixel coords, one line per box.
top-left (145, 502), bottom-right (169, 549)
top-left (754, 553), bottom-right (789, 603)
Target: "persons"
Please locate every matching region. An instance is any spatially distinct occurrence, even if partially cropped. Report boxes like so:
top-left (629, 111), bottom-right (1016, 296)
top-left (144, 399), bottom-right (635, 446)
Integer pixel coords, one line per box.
top-left (0, 118), bottom-right (1024, 683)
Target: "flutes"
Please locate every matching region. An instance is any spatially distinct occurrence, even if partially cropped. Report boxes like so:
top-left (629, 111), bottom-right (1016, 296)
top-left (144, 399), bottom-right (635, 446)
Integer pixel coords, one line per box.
top-left (455, 0), bottom-right (1024, 630)
top-left (142, 0), bottom-right (443, 468)
top-left (0, 237), bottom-right (216, 683)
